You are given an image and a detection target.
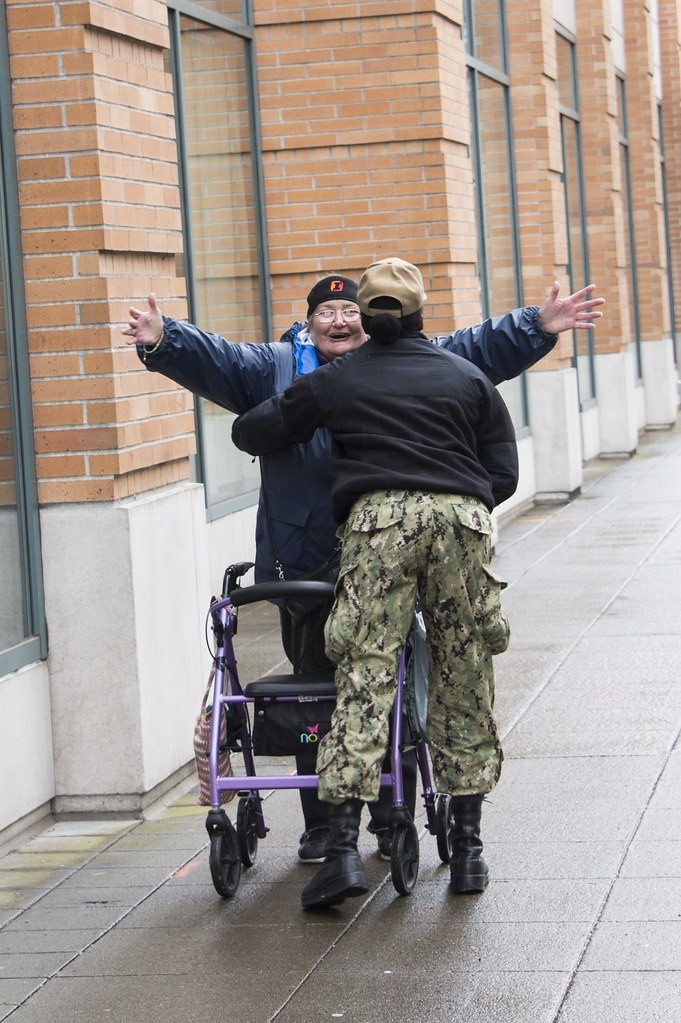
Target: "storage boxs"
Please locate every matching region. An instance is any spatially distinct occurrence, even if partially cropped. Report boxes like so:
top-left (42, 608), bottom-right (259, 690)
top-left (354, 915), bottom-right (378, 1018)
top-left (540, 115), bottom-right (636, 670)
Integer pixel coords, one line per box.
top-left (244, 673), bottom-right (338, 755)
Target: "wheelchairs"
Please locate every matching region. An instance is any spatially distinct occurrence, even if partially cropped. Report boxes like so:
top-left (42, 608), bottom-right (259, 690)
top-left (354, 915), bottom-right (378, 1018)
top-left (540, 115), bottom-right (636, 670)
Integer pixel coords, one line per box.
top-left (204, 554), bottom-right (456, 898)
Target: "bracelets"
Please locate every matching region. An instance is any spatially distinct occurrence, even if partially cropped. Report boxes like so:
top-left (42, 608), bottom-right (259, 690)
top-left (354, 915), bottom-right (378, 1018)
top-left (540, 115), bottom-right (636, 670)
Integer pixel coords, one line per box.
top-left (143, 330), bottom-right (164, 353)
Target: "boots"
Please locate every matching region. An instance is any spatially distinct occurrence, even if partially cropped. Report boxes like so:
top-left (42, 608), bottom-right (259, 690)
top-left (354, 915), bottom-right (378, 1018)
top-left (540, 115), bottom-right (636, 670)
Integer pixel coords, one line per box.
top-left (450, 795), bottom-right (489, 893)
top-left (301, 800), bottom-right (368, 909)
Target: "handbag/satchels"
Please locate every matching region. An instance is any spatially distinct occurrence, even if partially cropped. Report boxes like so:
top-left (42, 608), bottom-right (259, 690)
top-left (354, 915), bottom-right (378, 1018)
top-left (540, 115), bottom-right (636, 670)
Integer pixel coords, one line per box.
top-left (193, 703), bottom-right (236, 806)
top-left (285, 550), bottom-right (341, 678)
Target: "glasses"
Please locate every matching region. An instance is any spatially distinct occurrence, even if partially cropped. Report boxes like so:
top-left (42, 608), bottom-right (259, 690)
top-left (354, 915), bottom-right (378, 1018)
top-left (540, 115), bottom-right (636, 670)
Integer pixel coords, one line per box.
top-left (310, 307), bottom-right (361, 324)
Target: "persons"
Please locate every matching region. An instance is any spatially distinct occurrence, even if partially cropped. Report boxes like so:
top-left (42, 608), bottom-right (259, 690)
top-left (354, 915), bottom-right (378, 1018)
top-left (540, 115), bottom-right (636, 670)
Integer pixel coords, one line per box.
top-left (120, 273), bottom-right (605, 863)
top-left (230, 256), bottom-right (519, 909)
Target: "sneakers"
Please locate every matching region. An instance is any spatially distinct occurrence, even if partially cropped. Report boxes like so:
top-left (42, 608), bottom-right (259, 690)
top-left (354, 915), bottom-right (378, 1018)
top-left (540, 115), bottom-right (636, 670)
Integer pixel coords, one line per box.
top-left (298, 827), bottom-right (330, 864)
top-left (378, 831), bottom-right (392, 861)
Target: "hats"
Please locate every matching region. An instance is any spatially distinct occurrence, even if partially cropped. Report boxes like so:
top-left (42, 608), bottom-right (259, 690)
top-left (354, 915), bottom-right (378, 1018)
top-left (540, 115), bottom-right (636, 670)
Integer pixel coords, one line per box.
top-left (357, 257), bottom-right (427, 319)
top-left (306, 275), bottom-right (359, 317)
